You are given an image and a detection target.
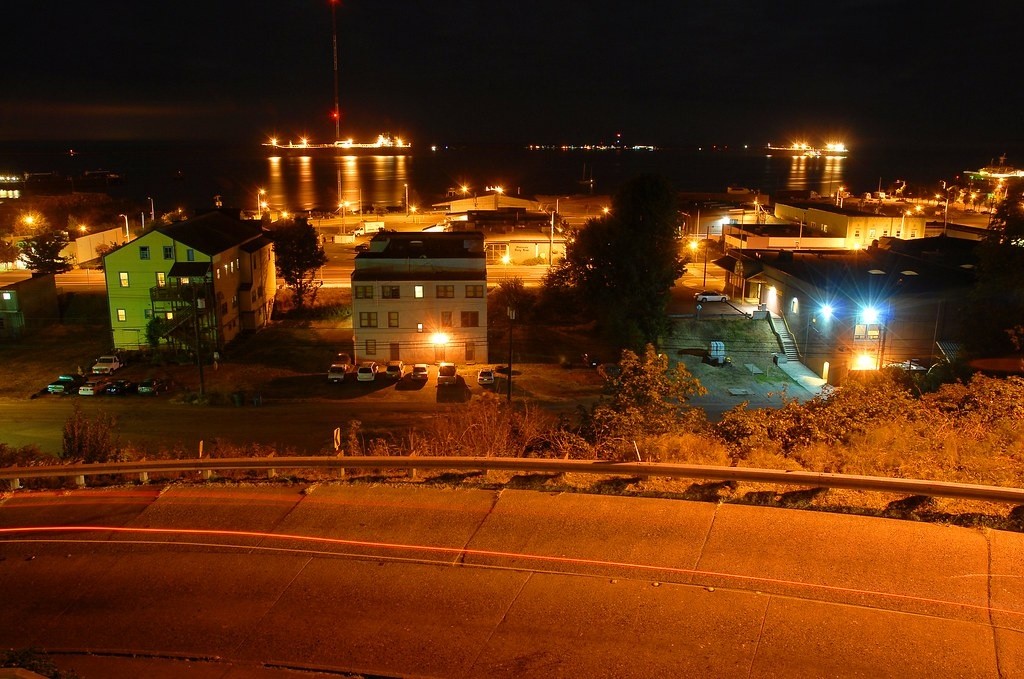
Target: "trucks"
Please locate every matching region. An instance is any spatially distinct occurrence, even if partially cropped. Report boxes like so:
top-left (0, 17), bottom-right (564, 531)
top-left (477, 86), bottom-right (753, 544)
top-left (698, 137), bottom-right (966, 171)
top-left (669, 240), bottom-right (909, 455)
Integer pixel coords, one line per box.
top-left (353, 221), bottom-right (384, 236)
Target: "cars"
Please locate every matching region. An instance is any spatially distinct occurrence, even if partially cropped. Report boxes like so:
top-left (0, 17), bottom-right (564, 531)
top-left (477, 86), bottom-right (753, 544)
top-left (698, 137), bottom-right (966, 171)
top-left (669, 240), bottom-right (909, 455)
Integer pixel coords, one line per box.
top-left (694, 290), bottom-right (730, 304)
top-left (478, 368), bottom-right (495, 386)
top-left (334, 353), bottom-right (351, 366)
top-left (80, 380), bottom-right (104, 395)
top-left (411, 364), bottom-right (429, 380)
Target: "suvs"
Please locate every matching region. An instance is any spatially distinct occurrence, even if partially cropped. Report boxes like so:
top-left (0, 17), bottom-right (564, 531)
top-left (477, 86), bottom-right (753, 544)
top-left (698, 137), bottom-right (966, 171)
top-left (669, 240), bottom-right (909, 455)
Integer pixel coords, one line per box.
top-left (327, 363), bottom-right (347, 384)
top-left (581, 352), bottom-right (598, 369)
top-left (437, 362), bottom-right (458, 384)
top-left (600, 363), bottom-right (617, 383)
top-left (385, 361), bottom-right (405, 380)
top-left (139, 377), bottom-right (169, 397)
top-left (357, 360), bottom-right (378, 382)
top-left (106, 379), bottom-right (131, 395)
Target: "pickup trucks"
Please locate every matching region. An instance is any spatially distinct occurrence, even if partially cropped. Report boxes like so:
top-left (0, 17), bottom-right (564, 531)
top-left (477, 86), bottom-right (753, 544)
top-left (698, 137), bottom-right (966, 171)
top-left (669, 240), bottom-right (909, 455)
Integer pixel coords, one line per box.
top-left (48, 373), bottom-right (78, 395)
top-left (91, 355), bottom-right (123, 376)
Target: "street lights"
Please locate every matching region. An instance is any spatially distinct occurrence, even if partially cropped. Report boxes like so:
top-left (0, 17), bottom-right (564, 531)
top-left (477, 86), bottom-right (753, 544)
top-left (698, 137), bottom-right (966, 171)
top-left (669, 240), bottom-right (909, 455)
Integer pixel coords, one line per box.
top-left (411, 205), bottom-right (417, 221)
top-left (256, 188), bottom-right (290, 223)
top-left (704, 224), bottom-right (713, 290)
top-left (148, 195), bottom-right (155, 220)
top-left (507, 298), bottom-right (515, 402)
top-left (121, 214), bottom-right (129, 244)
top-left (549, 208), bottom-right (557, 269)
top-left (339, 200), bottom-right (348, 235)
